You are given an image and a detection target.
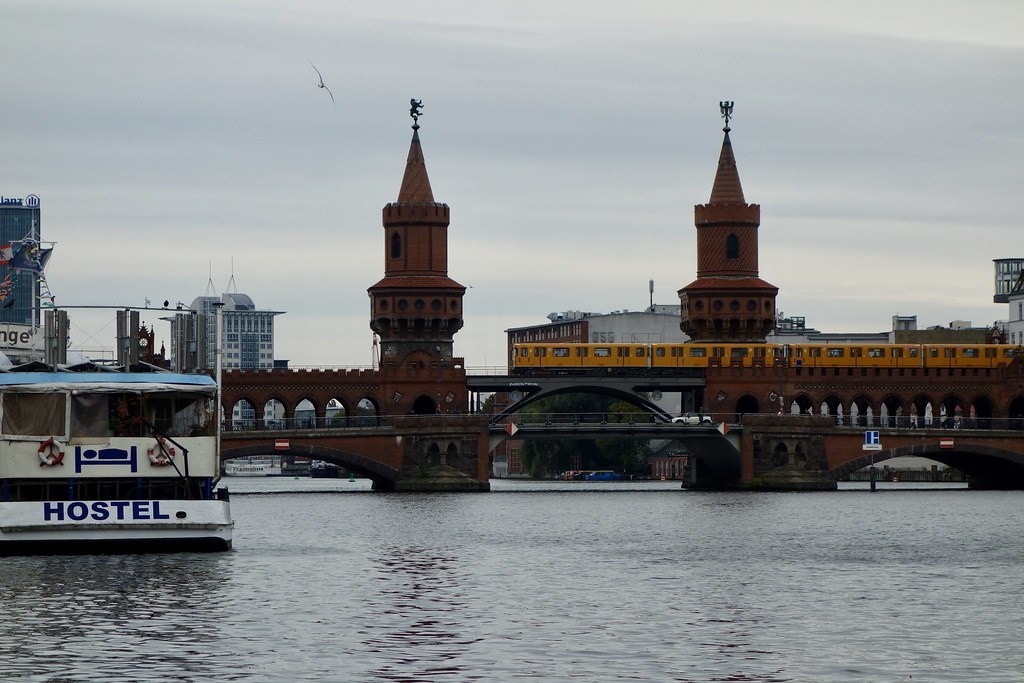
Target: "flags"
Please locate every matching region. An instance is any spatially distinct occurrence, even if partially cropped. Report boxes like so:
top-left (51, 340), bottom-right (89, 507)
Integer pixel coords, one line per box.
top-left (0, 242), bottom-right (52, 303)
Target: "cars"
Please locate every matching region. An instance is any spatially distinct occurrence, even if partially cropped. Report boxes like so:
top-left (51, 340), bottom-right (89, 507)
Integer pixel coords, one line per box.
top-left (560, 471), bottom-right (623, 481)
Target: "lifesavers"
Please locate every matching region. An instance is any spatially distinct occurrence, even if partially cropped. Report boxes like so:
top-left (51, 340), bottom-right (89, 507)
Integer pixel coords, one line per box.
top-left (147, 438), bottom-right (175, 465)
top-left (39, 439), bottom-right (65, 465)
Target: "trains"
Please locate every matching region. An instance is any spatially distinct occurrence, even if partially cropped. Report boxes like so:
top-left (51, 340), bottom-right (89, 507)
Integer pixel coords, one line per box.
top-left (511, 339), bottom-right (1024, 379)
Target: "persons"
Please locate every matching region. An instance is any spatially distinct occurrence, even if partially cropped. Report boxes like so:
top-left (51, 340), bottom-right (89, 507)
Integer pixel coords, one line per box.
top-left (436, 404), bottom-right (442, 414)
top-left (696, 406), bottom-right (704, 426)
top-left (406, 407), bottom-right (415, 414)
top-left (384, 348), bottom-right (390, 355)
top-left (451, 406), bottom-right (460, 415)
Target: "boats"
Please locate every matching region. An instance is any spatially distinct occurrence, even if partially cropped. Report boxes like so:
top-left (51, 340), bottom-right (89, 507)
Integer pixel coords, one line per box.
top-left (0, 359), bottom-right (233, 550)
top-left (225, 463), bottom-right (268, 477)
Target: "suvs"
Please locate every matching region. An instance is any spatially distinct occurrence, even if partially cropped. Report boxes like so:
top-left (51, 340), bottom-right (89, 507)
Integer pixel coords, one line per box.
top-left (670, 411), bottom-right (712, 425)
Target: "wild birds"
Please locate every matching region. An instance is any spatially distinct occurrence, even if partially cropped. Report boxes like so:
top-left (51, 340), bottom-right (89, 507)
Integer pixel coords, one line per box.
top-left (469, 285), bottom-right (475, 288)
top-left (306, 58), bottom-right (334, 103)
